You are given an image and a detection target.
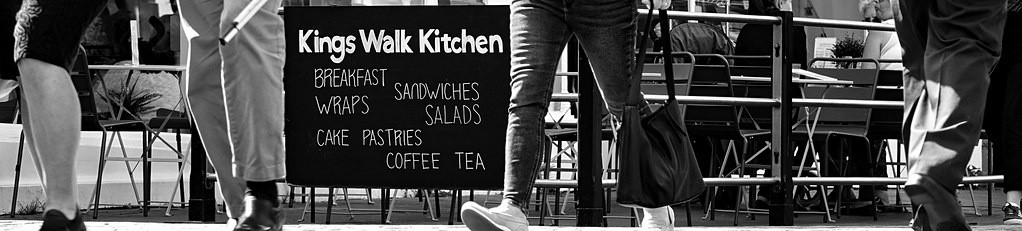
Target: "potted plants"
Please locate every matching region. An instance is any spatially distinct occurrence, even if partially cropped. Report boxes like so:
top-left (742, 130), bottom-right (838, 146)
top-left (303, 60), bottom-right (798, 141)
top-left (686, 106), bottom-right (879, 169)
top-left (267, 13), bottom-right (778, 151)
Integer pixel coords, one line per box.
top-left (827, 31), bottom-right (865, 69)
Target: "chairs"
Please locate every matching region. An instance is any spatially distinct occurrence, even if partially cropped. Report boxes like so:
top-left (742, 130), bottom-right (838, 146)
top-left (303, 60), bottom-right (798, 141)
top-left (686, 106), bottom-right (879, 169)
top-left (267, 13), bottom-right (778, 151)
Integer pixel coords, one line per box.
top-left (534, 51), bottom-right (903, 223)
top-left (149, 115), bottom-right (189, 212)
top-left (11, 44), bottom-right (149, 219)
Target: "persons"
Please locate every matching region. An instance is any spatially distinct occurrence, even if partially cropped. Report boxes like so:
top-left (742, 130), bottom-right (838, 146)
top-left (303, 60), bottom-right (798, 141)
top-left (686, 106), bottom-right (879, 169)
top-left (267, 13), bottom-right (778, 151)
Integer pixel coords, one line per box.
top-left (732, 0), bottom-right (808, 211)
top-left (891, 0), bottom-right (1007, 231)
top-left (0, 0), bottom-right (103, 231)
top-left (859, 0), bottom-right (886, 32)
top-left (177, 0), bottom-right (285, 231)
top-left (461, 1), bottom-right (675, 231)
top-left (983, 0), bottom-right (1022, 226)
top-left (809, 1), bottom-right (904, 210)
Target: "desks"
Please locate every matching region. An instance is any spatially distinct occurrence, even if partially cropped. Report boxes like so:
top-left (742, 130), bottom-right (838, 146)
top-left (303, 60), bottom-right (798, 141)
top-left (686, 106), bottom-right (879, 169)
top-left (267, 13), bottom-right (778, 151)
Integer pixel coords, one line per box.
top-left (82, 64), bottom-right (189, 217)
top-left (703, 78), bottom-right (853, 223)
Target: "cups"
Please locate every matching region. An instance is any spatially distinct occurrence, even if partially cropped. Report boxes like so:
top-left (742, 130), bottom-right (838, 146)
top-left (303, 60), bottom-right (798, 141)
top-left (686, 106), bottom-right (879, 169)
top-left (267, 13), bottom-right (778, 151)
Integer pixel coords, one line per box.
top-left (792, 63), bottom-right (801, 81)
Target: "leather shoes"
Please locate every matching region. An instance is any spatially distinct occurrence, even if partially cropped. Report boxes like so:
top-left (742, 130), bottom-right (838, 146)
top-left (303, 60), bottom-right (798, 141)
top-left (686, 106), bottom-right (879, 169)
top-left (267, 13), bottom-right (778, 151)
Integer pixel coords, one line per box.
top-left (233, 195), bottom-right (286, 231)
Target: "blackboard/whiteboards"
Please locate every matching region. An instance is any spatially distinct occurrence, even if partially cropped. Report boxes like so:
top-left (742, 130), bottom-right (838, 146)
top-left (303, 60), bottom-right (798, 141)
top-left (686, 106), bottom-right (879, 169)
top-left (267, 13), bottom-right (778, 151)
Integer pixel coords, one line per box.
top-left (281, 3), bottom-right (511, 190)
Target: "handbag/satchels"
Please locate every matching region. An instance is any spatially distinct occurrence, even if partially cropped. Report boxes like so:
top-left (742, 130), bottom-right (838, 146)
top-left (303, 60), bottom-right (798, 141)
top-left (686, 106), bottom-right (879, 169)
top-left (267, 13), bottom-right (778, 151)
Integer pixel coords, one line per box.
top-left (616, 0), bottom-right (708, 210)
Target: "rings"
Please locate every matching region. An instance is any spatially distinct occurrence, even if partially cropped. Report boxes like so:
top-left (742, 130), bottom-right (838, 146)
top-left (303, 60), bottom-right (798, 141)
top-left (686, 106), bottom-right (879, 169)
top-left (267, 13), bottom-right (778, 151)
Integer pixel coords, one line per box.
top-left (667, 2), bottom-right (670, 6)
top-left (662, 2), bottom-right (667, 6)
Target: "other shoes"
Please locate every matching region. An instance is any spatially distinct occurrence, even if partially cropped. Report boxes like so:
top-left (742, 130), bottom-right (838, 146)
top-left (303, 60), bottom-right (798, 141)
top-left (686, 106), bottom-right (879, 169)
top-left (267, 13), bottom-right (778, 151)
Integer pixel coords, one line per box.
top-left (460, 198), bottom-right (530, 231)
top-left (39, 203), bottom-right (87, 231)
top-left (642, 206), bottom-right (676, 231)
top-left (1002, 201), bottom-right (1022, 224)
top-left (903, 173), bottom-right (973, 231)
